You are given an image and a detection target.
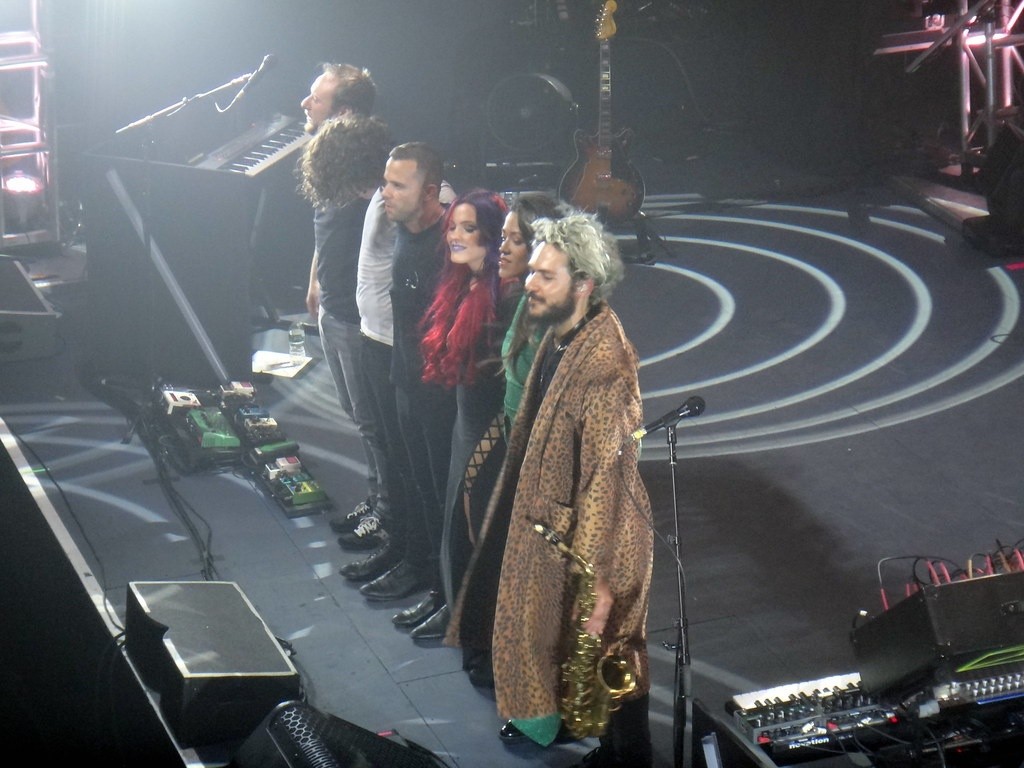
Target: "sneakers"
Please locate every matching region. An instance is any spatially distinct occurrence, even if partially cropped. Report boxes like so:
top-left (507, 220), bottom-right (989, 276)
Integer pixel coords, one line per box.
top-left (338, 516), bottom-right (391, 550)
top-left (330, 497), bottom-right (371, 533)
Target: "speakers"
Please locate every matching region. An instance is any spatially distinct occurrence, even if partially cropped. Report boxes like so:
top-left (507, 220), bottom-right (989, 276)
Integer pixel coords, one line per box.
top-left (0, 253), bottom-right (57, 366)
top-left (125, 580), bottom-right (301, 749)
top-left (223, 700), bottom-right (451, 768)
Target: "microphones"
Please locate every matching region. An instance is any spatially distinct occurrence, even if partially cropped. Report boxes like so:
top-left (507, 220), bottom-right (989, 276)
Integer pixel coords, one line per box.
top-left (621, 395), bottom-right (706, 445)
top-left (234, 52), bottom-right (277, 102)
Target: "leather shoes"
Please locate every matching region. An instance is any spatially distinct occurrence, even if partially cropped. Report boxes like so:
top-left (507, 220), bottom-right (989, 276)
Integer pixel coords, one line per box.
top-left (499, 719), bottom-right (573, 741)
top-left (410, 602), bottom-right (449, 639)
top-left (340, 541), bottom-right (402, 578)
top-left (391, 589), bottom-right (446, 626)
top-left (359, 559), bottom-right (425, 600)
top-left (471, 665), bottom-right (496, 688)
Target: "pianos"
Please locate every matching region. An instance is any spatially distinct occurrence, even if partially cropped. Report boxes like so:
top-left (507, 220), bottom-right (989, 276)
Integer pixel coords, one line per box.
top-left (78, 97), bottom-right (313, 396)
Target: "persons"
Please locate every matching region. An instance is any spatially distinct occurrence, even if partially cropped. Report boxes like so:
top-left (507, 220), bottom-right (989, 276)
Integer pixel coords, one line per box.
top-left (300, 60), bottom-right (653, 767)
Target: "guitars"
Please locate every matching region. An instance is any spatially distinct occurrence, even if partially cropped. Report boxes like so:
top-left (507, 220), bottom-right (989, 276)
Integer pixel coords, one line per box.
top-left (555, 0), bottom-right (647, 226)
